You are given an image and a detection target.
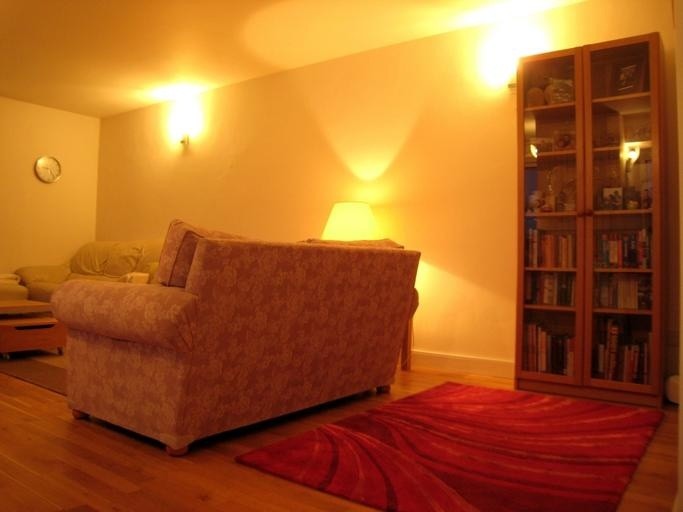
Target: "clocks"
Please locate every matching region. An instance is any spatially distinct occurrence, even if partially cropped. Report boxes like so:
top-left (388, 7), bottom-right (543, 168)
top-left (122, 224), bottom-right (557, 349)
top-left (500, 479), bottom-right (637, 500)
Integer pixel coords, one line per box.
top-left (34, 155), bottom-right (62, 184)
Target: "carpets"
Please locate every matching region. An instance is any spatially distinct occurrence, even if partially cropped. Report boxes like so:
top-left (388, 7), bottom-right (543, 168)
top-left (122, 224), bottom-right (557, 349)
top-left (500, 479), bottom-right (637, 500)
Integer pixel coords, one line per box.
top-left (236, 380), bottom-right (665, 512)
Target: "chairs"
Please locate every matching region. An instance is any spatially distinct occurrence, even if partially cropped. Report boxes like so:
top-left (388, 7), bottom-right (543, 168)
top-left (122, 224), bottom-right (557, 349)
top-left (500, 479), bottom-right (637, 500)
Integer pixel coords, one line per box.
top-left (0, 272), bottom-right (29, 301)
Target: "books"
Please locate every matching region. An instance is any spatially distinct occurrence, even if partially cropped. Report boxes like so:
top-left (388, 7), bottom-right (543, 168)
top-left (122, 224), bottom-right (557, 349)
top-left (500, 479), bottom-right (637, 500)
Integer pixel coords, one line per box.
top-left (521, 220), bottom-right (654, 385)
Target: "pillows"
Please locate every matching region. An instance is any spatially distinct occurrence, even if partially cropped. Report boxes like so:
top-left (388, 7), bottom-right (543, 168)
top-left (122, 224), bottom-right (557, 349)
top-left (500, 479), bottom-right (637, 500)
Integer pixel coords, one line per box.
top-left (148, 219), bottom-right (245, 288)
top-left (69, 240), bottom-right (107, 275)
top-left (105, 243), bottom-right (142, 278)
top-left (115, 272), bottom-right (150, 286)
top-left (135, 242), bottom-right (163, 274)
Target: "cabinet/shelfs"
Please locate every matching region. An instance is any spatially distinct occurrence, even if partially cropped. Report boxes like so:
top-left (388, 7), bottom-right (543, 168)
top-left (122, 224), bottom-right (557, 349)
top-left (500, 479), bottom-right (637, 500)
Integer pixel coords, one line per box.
top-left (516, 32), bottom-right (671, 408)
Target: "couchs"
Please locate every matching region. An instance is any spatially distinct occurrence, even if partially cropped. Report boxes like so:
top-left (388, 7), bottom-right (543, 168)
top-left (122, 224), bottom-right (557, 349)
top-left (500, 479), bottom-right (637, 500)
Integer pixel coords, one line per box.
top-left (51, 239), bottom-right (420, 457)
top-left (16, 240), bottom-right (163, 301)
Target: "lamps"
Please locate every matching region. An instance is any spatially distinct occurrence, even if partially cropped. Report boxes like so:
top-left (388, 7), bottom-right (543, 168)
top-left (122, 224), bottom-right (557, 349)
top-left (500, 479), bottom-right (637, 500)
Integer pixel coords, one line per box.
top-left (321, 202), bottom-right (378, 241)
top-left (169, 113), bottom-right (204, 167)
